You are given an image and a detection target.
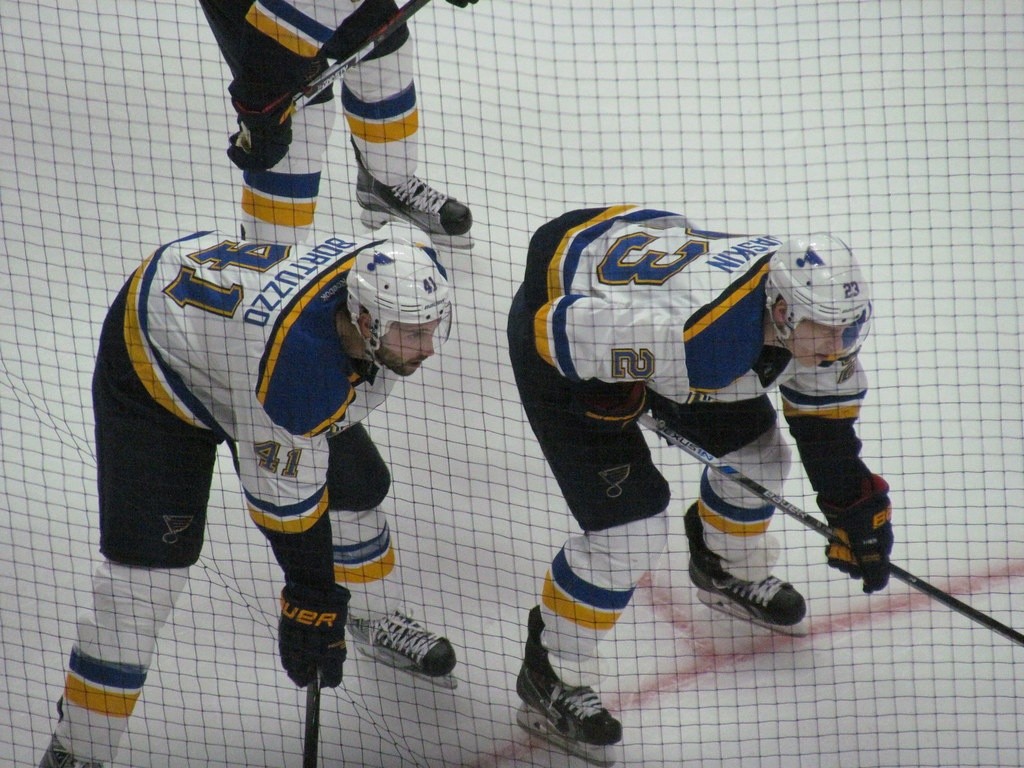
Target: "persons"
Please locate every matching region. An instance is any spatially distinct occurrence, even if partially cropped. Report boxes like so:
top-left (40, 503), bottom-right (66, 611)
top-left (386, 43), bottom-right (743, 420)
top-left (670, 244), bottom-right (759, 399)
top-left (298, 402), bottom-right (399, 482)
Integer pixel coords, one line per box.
top-left (194, 1), bottom-right (482, 259)
top-left (505, 201), bottom-right (895, 768)
top-left (35, 216), bottom-right (461, 768)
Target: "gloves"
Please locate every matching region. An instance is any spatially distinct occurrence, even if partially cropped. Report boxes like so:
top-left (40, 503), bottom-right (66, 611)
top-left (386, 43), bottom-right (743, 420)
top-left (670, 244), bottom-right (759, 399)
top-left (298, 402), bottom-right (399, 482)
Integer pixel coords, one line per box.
top-left (585, 382), bottom-right (654, 431)
top-left (278, 580), bottom-right (350, 689)
top-left (816, 486), bottom-right (898, 592)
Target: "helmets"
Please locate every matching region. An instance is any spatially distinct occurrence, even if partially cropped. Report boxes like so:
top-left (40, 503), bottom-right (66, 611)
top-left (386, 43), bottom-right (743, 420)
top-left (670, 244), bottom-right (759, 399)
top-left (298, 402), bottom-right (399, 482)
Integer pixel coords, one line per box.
top-left (766, 233), bottom-right (868, 339)
top-left (345, 244), bottom-right (450, 348)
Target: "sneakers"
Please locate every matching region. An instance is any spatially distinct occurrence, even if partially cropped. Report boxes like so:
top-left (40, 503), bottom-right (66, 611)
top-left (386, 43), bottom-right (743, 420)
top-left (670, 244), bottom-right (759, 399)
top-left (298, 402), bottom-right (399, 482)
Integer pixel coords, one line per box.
top-left (683, 501), bottom-right (808, 638)
top-left (516, 605), bottom-right (624, 768)
top-left (357, 161), bottom-right (474, 252)
top-left (342, 609), bottom-right (459, 691)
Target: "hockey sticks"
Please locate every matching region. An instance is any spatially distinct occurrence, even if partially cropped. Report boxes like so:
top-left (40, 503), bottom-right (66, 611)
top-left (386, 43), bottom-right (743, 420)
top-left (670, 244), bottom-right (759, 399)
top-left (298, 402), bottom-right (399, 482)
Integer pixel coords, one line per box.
top-left (636, 412), bottom-right (1024, 648)
top-left (239, 0), bottom-right (430, 151)
top-left (301, 666), bottom-right (322, 768)
